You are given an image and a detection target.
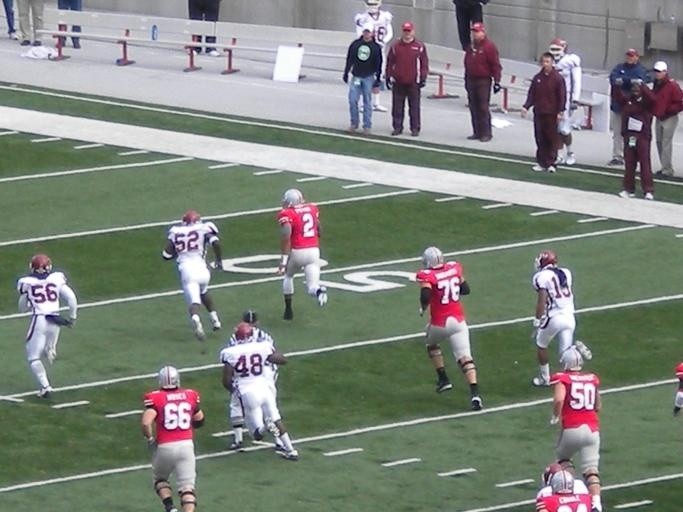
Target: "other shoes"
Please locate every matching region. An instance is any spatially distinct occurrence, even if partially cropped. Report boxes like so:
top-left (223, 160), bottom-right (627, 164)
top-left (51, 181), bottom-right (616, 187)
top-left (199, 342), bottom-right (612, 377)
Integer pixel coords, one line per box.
top-left (547, 165), bottom-right (556, 173)
top-left (480, 135), bottom-right (490, 142)
top-left (656, 170), bottom-right (672, 179)
top-left (348, 126), bottom-right (359, 134)
top-left (411, 128), bottom-right (418, 136)
top-left (373, 104), bottom-right (388, 112)
top-left (644, 192), bottom-right (653, 200)
top-left (363, 127), bottom-right (372, 137)
top-left (565, 153), bottom-right (576, 165)
top-left (467, 134), bottom-right (480, 140)
top-left (360, 104), bottom-right (364, 111)
top-left (607, 159), bottom-right (624, 166)
top-left (618, 190), bottom-right (635, 198)
top-left (391, 129), bottom-right (402, 135)
top-left (554, 155), bottom-right (565, 164)
top-left (532, 165), bottom-right (546, 172)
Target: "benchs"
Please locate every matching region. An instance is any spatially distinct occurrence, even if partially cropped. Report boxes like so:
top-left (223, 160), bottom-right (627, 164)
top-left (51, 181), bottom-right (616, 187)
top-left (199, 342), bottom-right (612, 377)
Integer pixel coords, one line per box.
top-left (213, 19), bottom-right (365, 84)
top-left (31, 6), bottom-right (213, 77)
top-left (417, 36), bottom-right (613, 132)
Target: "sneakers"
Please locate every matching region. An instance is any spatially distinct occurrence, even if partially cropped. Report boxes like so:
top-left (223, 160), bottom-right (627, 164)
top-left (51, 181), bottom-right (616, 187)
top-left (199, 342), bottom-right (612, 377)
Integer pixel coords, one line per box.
top-left (186, 46), bottom-right (222, 59)
top-left (281, 302), bottom-right (294, 321)
top-left (262, 415), bottom-right (282, 438)
top-left (55, 40), bottom-right (82, 51)
top-left (573, 338), bottom-right (592, 362)
top-left (315, 285), bottom-right (330, 308)
top-left (471, 393), bottom-right (485, 412)
top-left (20, 39), bottom-right (43, 48)
top-left (208, 315), bottom-right (223, 332)
top-left (191, 317), bottom-right (207, 341)
top-left (434, 378), bottom-right (455, 394)
top-left (273, 444), bottom-right (300, 461)
top-left (6, 30), bottom-right (20, 41)
top-left (591, 501), bottom-right (603, 512)
top-left (34, 384), bottom-right (55, 398)
top-left (228, 440), bottom-right (244, 453)
top-left (531, 374), bottom-right (551, 388)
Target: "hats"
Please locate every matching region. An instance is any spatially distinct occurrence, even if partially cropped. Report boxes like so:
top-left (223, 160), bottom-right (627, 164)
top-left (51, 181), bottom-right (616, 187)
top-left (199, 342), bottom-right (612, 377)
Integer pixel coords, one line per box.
top-left (241, 309), bottom-right (259, 324)
top-left (400, 21), bottom-right (415, 32)
top-left (630, 77), bottom-right (645, 88)
top-left (653, 61), bottom-right (668, 73)
top-left (623, 47), bottom-right (640, 59)
top-left (469, 22), bottom-right (487, 33)
top-left (361, 22), bottom-right (375, 34)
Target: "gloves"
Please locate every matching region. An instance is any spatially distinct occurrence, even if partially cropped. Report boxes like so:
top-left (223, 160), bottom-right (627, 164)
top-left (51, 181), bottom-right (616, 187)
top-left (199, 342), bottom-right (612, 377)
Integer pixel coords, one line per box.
top-left (532, 317), bottom-right (543, 330)
top-left (384, 78), bottom-right (392, 90)
top-left (66, 318), bottom-right (77, 329)
top-left (374, 74), bottom-right (381, 84)
top-left (418, 80), bottom-right (426, 89)
top-left (145, 437), bottom-right (158, 454)
top-left (549, 414), bottom-right (561, 426)
top-left (673, 407), bottom-right (681, 418)
top-left (212, 260), bottom-right (224, 272)
top-left (340, 72), bottom-right (350, 85)
top-left (491, 82), bottom-right (503, 93)
top-left (274, 262), bottom-right (287, 276)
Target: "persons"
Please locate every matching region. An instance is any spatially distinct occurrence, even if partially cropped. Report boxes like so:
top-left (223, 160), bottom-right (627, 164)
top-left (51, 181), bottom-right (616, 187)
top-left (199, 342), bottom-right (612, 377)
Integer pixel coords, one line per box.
top-left (164, 210), bottom-right (223, 342)
top-left (416, 246), bottom-right (483, 411)
top-left (275, 189), bottom-right (328, 320)
top-left (140, 310), bottom-right (298, 512)
top-left (2, 0), bottom-right (18, 40)
top-left (16, 0), bottom-right (46, 46)
top-left (17, 254), bottom-right (77, 398)
top-left (188, 0), bottom-right (221, 56)
top-left (57, 0), bottom-right (82, 49)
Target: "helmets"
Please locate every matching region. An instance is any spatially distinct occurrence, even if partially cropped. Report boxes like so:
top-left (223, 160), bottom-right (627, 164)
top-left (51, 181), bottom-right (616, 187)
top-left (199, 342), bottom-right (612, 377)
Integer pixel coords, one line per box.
top-left (233, 321), bottom-right (254, 341)
top-left (422, 245), bottom-right (444, 269)
top-left (550, 468), bottom-right (576, 495)
top-left (181, 209), bottom-right (201, 225)
top-left (27, 251), bottom-right (54, 276)
top-left (280, 186), bottom-right (306, 209)
top-left (363, 0), bottom-right (384, 14)
top-left (549, 37), bottom-right (569, 57)
top-left (533, 249), bottom-right (559, 270)
top-left (558, 347), bottom-right (585, 372)
top-left (156, 364), bottom-right (182, 391)
top-left (541, 461), bottom-right (563, 486)
